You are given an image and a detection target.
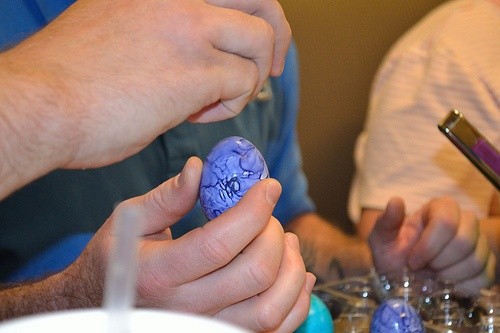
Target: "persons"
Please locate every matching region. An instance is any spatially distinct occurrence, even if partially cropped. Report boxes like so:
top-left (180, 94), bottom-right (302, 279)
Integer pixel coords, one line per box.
top-left (0, 0), bottom-right (495, 332)
top-left (348, 0), bottom-right (499, 245)
